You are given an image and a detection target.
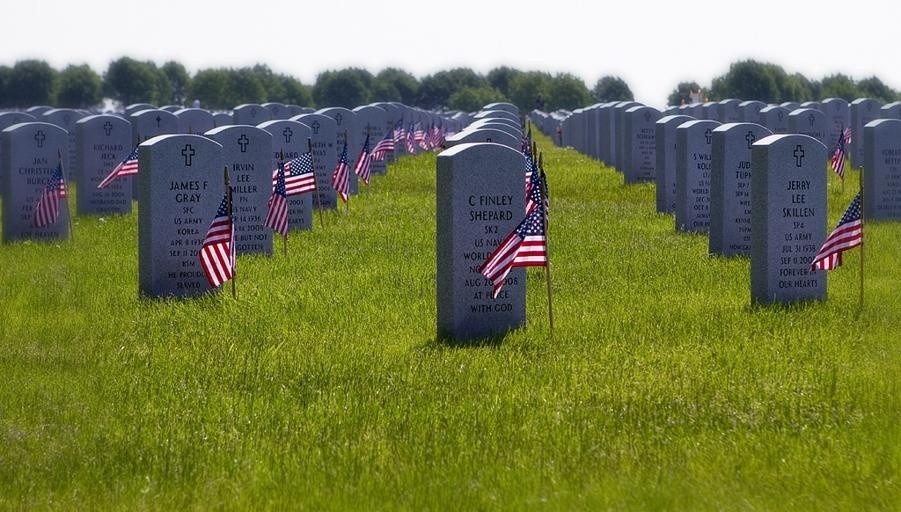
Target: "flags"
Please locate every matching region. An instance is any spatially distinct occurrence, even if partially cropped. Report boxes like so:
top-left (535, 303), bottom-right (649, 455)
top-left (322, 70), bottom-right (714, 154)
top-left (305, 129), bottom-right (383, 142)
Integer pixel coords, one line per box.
top-left (525, 137), bottom-right (533, 171)
top-left (429, 128), bottom-right (434, 148)
top-left (435, 127), bottom-right (444, 147)
top-left (526, 152), bottom-right (546, 214)
top-left (810, 165), bottom-right (863, 297)
top-left (33, 161), bottom-right (73, 237)
top-left (331, 130), bottom-right (350, 211)
top-left (355, 133), bottom-right (371, 187)
top-left (406, 121), bottom-right (414, 154)
top-left (97, 143), bottom-right (139, 191)
top-left (525, 128), bottom-right (531, 151)
top-left (272, 138), bottom-right (325, 230)
top-left (262, 149), bottom-right (288, 256)
top-left (831, 130), bottom-right (844, 193)
top-left (199, 166), bottom-right (237, 299)
top-left (843, 127), bottom-right (852, 144)
top-left (420, 129), bottom-right (431, 150)
top-left (370, 129), bottom-right (396, 174)
top-left (394, 119), bottom-right (406, 143)
top-left (478, 179), bottom-right (553, 330)
top-left (522, 142), bottom-right (539, 196)
top-left (413, 121), bottom-right (423, 140)
